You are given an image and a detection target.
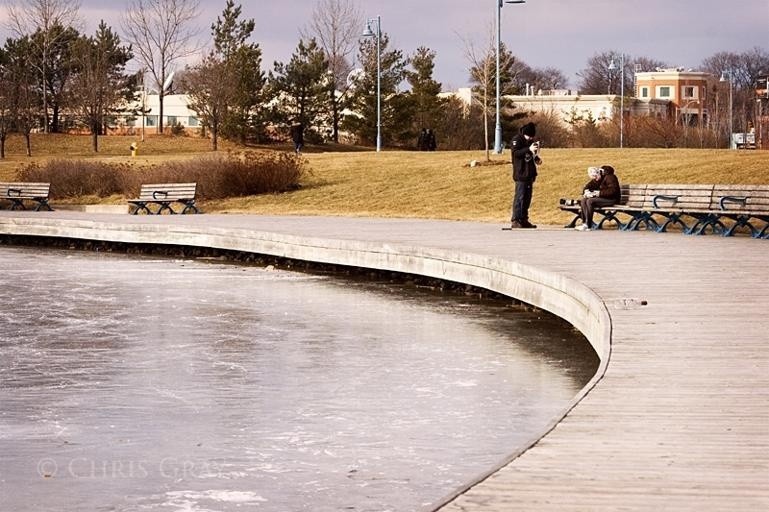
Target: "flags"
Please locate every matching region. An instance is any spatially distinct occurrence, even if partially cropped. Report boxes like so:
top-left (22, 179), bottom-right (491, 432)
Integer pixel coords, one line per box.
top-left (605, 55), bottom-right (622, 72)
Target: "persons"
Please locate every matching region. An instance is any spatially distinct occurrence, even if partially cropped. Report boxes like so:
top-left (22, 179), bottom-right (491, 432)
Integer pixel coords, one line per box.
top-left (511, 123), bottom-right (542, 228)
top-left (418, 128), bottom-right (436, 151)
top-left (575, 166), bottom-right (621, 230)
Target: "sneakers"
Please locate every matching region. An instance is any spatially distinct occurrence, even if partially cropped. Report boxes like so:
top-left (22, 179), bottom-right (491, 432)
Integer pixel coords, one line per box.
top-left (574, 223), bottom-right (590, 231)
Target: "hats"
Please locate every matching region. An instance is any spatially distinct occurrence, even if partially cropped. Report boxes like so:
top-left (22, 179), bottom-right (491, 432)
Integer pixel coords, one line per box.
top-left (522, 123), bottom-right (535, 137)
top-left (588, 167), bottom-right (599, 179)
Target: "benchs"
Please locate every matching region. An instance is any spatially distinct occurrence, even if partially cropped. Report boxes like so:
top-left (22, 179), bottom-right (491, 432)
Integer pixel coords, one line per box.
top-left (0, 182), bottom-right (53, 211)
top-left (127, 183), bottom-right (204, 214)
top-left (557, 184), bottom-right (769, 239)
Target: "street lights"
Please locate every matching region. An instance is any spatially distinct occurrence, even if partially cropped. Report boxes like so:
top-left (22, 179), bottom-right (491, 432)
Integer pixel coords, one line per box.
top-left (720, 66), bottom-right (734, 151)
top-left (362, 15), bottom-right (385, 151)
top-left (493, 0), bottom-right (526, 153)
top-left (609, 52), bottom-right (625, 149)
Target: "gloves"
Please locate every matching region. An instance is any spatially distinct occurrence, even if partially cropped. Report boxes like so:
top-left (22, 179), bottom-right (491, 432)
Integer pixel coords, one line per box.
top-left (583, 189), bottom-right (600, 198)
top-left (529, 143), bottom-right (537, 153)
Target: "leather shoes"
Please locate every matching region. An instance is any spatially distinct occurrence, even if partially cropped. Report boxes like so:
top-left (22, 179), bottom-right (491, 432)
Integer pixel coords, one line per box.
top-left (512, 221), bottom-right (536, 228)
